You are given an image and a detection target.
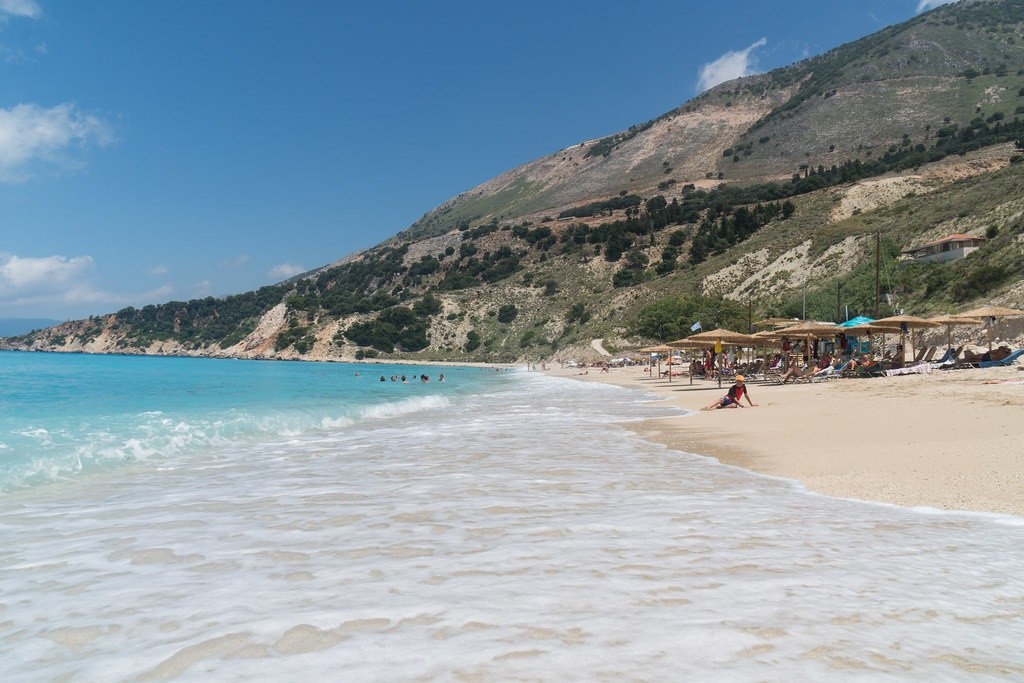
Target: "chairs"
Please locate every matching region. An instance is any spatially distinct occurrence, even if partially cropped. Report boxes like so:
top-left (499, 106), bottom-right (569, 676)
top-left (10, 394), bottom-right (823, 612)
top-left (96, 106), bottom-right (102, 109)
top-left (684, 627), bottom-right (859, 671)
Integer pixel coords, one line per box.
top-left (658, 344), bottom-right (1024, 385)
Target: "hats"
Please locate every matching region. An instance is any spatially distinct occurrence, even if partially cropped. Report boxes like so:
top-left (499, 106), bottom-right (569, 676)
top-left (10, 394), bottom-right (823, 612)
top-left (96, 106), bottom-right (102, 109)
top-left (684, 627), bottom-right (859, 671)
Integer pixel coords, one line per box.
top-left (810, 359), bottom-right (818, 363)
top-left (736, 375), bottom-right (745, 381)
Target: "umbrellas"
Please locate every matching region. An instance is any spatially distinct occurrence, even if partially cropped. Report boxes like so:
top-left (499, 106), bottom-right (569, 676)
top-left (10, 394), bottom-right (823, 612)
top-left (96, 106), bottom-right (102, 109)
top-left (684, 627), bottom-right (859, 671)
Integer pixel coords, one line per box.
top-left (928, 313), bottom-right (981, 351)
top-left (952, 304), bottom-right (1024, 355)
top-left (636, 338), bottom-right (679, 382)
top-left (667, 309), bottom-right (933, 368)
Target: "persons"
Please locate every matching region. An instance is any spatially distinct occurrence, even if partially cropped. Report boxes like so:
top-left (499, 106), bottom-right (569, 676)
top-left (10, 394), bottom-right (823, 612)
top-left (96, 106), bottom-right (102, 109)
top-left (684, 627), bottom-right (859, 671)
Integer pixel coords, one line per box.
top-left (699, 375), bottom-right (757, 411)
top-left (689, 336), bottom-right (906, 383)
top-left (456, 357), bottom-right (674, 376)
top-left (970, 346), bottom-right (1012, 364)
top-left (380, 372), bottom-right (445, 383)
